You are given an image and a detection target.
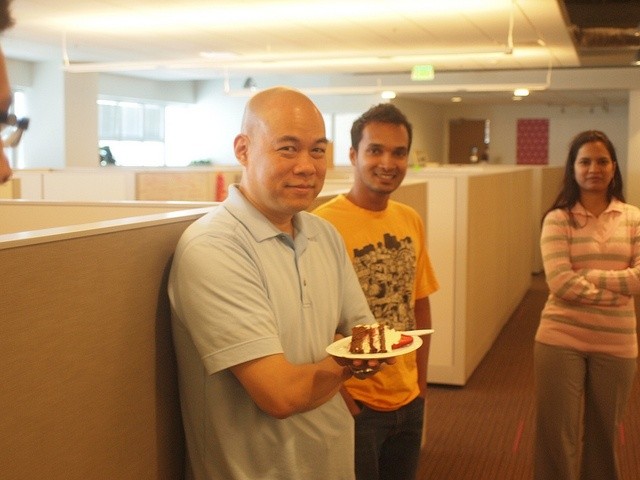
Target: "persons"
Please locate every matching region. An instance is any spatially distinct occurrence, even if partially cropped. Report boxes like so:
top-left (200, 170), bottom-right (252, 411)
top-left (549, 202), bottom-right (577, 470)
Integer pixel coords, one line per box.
top-left (166, 87), bottom-right (399, 480)
top-left (311, 103), bottom-right (441, 479)
top-left (0, 1), bottom-right (31, 188)
top-left (532, 130), bottom-right (640, 480)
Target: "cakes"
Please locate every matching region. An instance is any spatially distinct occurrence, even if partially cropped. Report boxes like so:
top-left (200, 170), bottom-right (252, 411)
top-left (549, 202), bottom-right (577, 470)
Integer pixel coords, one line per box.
top-left (349, 323), bottom-right (396, 355)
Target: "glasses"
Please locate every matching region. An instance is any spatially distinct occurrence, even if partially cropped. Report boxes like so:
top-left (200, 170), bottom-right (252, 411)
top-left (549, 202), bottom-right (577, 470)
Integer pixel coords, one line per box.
top-left (0, 114), bottom-right (30, 148)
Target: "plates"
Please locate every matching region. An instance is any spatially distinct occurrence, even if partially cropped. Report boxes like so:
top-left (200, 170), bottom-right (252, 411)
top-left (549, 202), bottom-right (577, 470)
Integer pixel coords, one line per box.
top-left (325, 332), bottom-right (423, 359)
